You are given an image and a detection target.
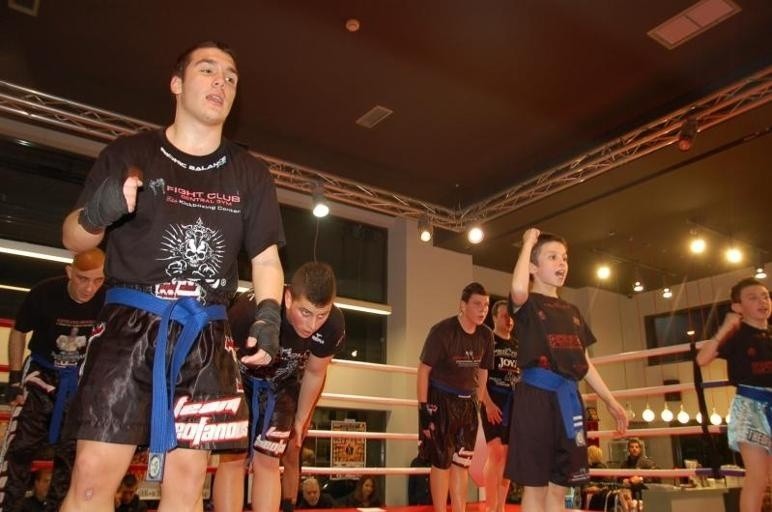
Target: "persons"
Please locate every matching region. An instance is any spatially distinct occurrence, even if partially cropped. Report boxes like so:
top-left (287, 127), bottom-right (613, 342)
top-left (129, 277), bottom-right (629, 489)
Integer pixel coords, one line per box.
top-left (209, 258), bottom-right (348, 512)
top-left (616, 439), bottom-right (659, 501)
top-left (21, 468), bottom-right (53, 511)
top-left (500, 228), bottom-right (629, 512)
top-left (300, 478), bottom-right (335, 509)
top-left (695, 275), bottom-right (772, 512)
top-left (58, 41), bottom-right (289, 512)
top-left (114, 474), bottom-right (148, 512)
top-left (345, 475), bottom-right (381, 507)
top-left (587, 445), bottom-right (608, 469)
top-left (415, 281), bottom-right (496, 512)
top-left (478, 298), bottom-right (521, 512)
top-left (0, 246), bottom-right (106, 512)
top-left (408, 457), bottom-right (451, 506)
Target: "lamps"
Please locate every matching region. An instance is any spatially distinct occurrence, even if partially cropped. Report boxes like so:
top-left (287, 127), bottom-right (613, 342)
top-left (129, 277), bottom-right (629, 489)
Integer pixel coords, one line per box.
top-left (417, 214), bottom-right (433, 244)
top-left (592, 216), bottom-right (770, 427)
top-left (312, 185), bottom-right (330, 218)
top-left (676, 113), bottom-right (701, 153)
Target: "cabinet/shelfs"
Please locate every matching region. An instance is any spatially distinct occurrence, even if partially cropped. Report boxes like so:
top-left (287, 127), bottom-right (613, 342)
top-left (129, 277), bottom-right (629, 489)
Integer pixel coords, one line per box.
top-left (637, 482), bottom-right (731, 512)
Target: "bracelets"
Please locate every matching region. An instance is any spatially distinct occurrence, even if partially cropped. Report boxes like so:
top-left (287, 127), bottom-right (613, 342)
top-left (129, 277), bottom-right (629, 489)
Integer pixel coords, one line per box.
top-left (9, 369), bottom-right (22, 384)
